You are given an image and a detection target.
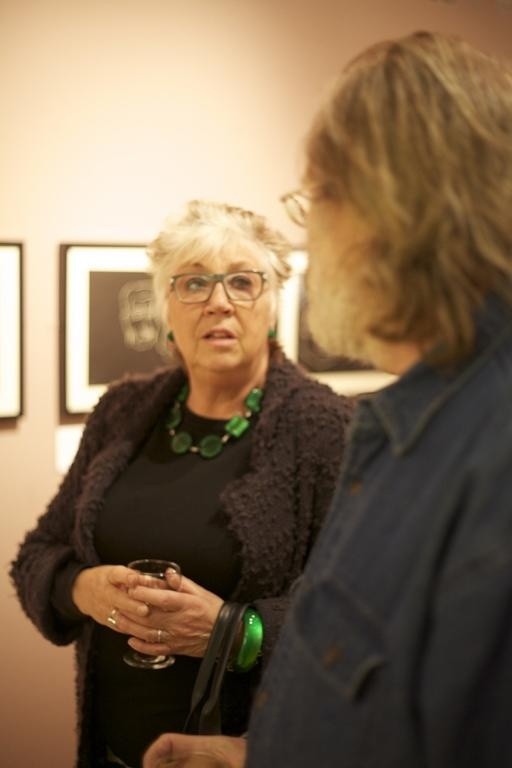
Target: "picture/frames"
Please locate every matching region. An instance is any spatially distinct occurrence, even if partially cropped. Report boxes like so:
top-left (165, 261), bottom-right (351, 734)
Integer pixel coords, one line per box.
top-left (57, 242), bottom-right (185, 418)
top-left (0, 239), bottom-right (26, 422)
top-left (271, 243), bottom-right (407, 400)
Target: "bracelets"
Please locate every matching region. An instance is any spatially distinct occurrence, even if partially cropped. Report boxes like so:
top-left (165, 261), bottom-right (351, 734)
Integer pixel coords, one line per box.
top-left (237, 609), bottom-right (264, 670)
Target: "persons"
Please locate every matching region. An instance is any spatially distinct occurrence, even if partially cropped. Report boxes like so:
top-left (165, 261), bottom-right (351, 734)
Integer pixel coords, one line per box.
top-left (141, 30), bottom-right (512, 768)
top-left (9, 199), bottom-right (356, 768)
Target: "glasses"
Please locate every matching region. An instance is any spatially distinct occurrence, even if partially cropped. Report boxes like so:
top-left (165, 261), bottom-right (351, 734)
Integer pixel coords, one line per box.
top-left (276, 176), bottom-right (354, 232)
top-left (164, 269), bottom-right (282, 307)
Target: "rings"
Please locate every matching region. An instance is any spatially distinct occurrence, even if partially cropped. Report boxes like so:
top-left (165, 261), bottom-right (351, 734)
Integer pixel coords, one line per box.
top-left (157, 630), bottom-right (162, 642)
top-left (107, 609), bottom-right (119, 625)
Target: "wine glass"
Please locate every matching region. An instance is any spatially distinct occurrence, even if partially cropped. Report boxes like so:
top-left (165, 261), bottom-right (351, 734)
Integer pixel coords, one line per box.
top-left (119, 560), bottom-right (182, 670)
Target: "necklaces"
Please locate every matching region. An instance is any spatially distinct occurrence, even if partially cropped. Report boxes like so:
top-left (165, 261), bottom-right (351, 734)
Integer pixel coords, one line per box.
top-left (165, 385), bottom-right (264, 458)
